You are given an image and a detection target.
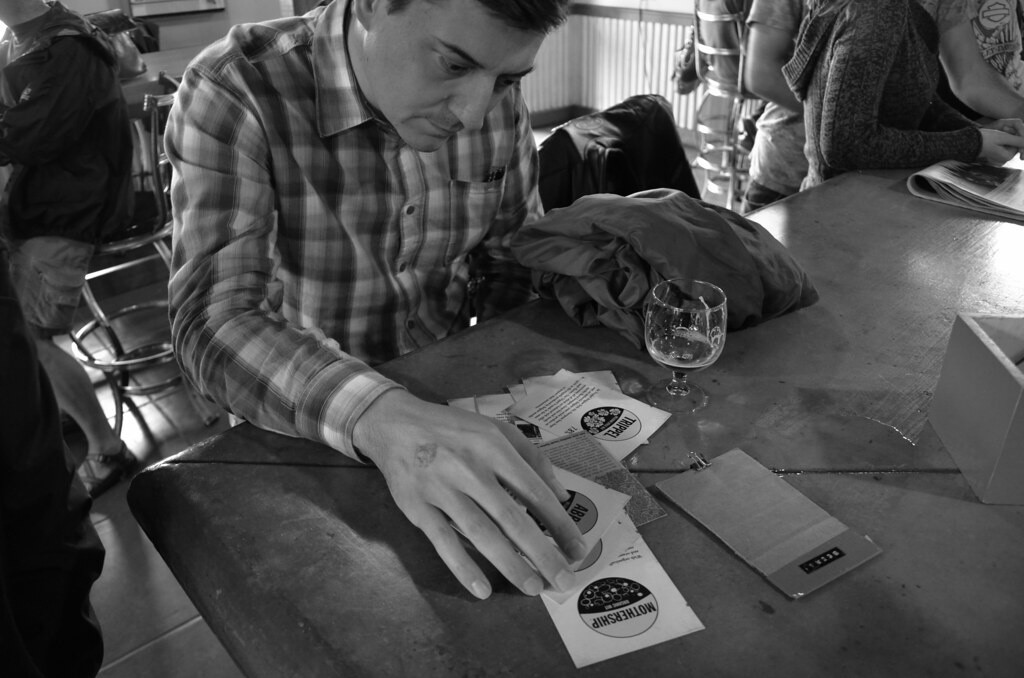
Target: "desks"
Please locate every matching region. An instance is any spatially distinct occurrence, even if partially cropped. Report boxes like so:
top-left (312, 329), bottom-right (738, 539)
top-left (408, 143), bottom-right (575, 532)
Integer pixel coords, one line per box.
top-left (123, 170), bottom-right (1024, 678)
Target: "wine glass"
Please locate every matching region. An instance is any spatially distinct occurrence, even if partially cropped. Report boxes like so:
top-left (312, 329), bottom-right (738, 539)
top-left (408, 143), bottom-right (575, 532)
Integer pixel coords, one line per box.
top-left (647, 277), bottom-right (728, 415)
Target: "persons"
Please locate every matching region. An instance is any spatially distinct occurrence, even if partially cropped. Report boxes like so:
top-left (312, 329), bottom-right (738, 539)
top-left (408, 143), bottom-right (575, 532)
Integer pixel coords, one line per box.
top-left (0, 0), bottom-right (138, 503)
top-left (163, 1), bottom-right (589, 601)
top-left (742, 0), bottom-right (1024, 216)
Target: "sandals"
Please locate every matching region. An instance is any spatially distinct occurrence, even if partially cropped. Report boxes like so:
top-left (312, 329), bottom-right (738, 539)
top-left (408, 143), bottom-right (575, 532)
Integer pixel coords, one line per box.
top-left (74, 439), bottom-right (138, 502)
